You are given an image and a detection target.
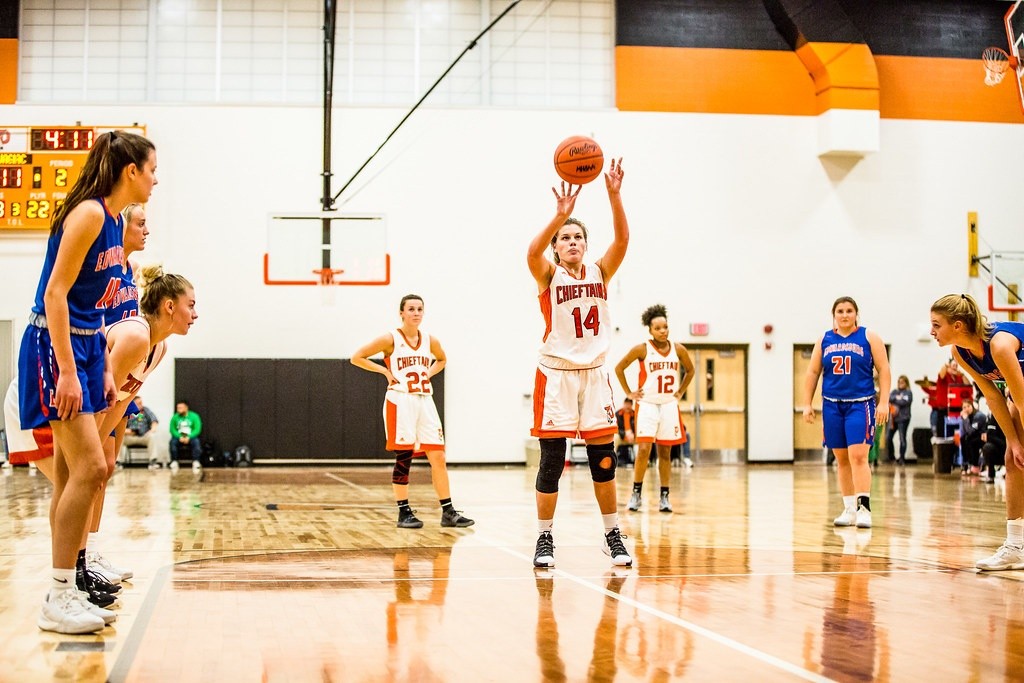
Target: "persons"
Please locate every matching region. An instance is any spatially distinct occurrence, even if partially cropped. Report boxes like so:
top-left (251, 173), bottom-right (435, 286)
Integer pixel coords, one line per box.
top-left (803, 296), bottom-right (892, 529)
top-left (350, 294), bottom-right (475, 528)
top-left (880, 357), bottom-right (1010, 485)
top-left (929, 293), bottom-right (1024, 571)
top-left (614, 397), bottom-right (694, 468)
top-left (167, 402), bottom-right (203, 468)
top-left (527, 157), bottom-right (633, 567)
top-left (615, 305), bottom-right (696, 512)
top-left (115, 395), bottom-right (161, 471)
top-left (4, 131), bottom-right (200, 635)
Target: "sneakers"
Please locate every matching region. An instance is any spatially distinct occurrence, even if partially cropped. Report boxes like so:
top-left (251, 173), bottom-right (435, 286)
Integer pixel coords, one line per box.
top-left (36, 548), bottom-right (133, 633)
top-left (834, 504), bottom-right (856, 525)
top-left (976, 541), bottom-right (1024, 570)
top-left (627, 489), bottom-right (642, 511)
top-left (659, 491), bottom-right (672, 512)
top-left (397, 507), bottom-right (423, 528)
top-left (533, 531), bottom-right (556, 567)
top-left (601, 527), bottom-right (632, 566)
top-left (440, 507), bottom-right (475, 527)
top-left (856, 505), bottom-right (871, 528)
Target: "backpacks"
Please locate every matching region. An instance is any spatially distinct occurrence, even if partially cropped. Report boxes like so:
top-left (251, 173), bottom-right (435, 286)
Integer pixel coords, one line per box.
top-left (200, 438), bottom-right (226, 467)
top-left (231, 445), bottom-right (254, 467)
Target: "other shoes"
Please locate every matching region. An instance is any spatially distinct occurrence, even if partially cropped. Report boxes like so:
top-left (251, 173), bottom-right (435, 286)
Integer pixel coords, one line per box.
top-left (622, 458), bottom-right (694, 468)
top-left (961, 465), bottom-right (1006, 477)
top-left (29, 462), bottom-right (37, 468)
top-left (170, 461), bottom-right (178, 468)
top-left (191, 461), bottom-right (201, 467)
top-left (148, 463), bottom-right (159, 470)
top-left (985, 479), bottom-right (995, 483)
top-left (1, 461), bottom-right (13, 467)
top-left (882, 458), bottom-right (905, 466)
top-left (115, 463), bottom-right (122, 469)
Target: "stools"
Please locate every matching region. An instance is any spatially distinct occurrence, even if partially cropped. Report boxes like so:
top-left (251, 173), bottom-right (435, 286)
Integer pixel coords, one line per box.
top-left (116, 436), bottom-right (147, 468)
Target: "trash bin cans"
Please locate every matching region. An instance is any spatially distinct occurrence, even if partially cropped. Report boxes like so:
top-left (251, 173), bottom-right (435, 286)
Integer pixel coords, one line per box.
top-left (932, 437), bottom-right (954, 474)
top-left (915, 428), bottom-right (933, 462)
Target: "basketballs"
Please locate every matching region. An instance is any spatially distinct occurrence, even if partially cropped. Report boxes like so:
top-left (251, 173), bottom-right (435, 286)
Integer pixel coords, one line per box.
top-left (552, 136), bottom-right (604, 185)
